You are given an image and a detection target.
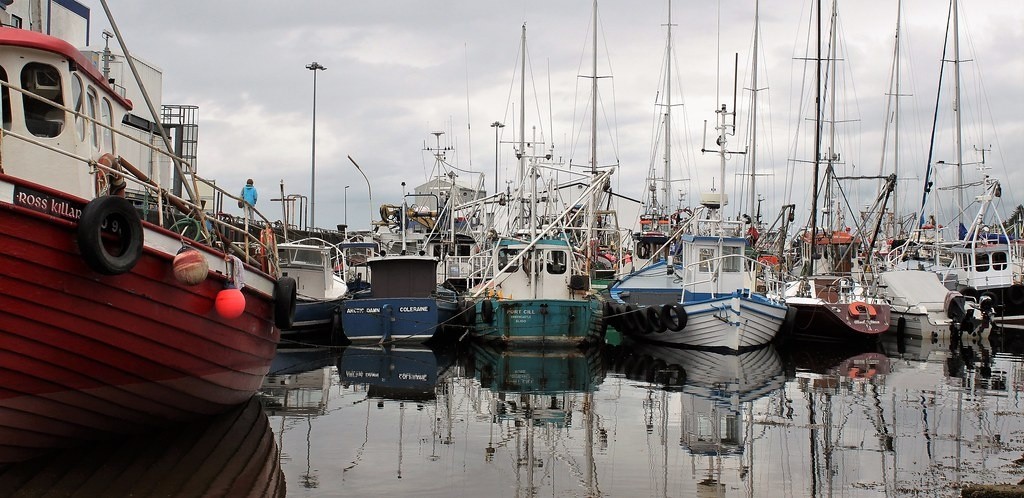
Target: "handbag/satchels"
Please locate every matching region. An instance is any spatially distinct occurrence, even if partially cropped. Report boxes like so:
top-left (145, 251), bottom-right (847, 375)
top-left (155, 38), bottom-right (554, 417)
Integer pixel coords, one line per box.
top-left (238, 188), bottom-right (244, 208)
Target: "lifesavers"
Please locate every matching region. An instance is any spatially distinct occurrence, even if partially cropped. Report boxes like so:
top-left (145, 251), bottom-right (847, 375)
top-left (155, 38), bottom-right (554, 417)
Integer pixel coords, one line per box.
top-left (616, 305), bottom-right (632, 335)
top-left (625, 304), bottom-right (639, 331)
top-left (274, 276), bottom-right (297, 329)
top-left (465, 301), bottom-right (476, 325)
top-left (481, 300), bottom-right (493, 323)
top-left (76, 194), bottom-right (146, 276)
top-left (634, 310), bottom-right (652, 335)
top-left (848, 302), bottom-right (877, 318)
top-left (646, 305), bottom-right (667, 334)
top-left (606, 301), bottom-right (615, 327)
top-left (662, 302), bottom-right (688, 332)
top-left (610, 304), bottom-right (621, 332)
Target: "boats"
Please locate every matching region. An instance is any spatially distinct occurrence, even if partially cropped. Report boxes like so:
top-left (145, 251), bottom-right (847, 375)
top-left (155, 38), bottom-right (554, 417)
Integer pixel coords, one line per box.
top-left (620, 334), bottom-right (788, 498)
top-left (468, 341), bottom-right (611, 498)
top-left (0, 0), bottom-right (300, 471)
top-left (0, 394), bottom-right (287, 498)
top-left (338, 343), bottom-right (438, 481)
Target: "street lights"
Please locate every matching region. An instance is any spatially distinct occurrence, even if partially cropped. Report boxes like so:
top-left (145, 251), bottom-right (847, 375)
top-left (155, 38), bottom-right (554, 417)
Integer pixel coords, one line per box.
top-left (304, 62), bottom-right (328, 232)
top-left (490, 121), bottom-right (506, 194)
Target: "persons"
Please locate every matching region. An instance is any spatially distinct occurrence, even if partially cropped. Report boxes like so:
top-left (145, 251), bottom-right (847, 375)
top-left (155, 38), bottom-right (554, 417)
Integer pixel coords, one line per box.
top-left (240, 179), bottom-right (258, 219)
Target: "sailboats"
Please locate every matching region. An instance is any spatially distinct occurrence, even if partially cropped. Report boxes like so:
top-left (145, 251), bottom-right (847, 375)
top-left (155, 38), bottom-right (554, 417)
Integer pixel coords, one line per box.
top-left (252, 0), bottom-right (1024, 351)
